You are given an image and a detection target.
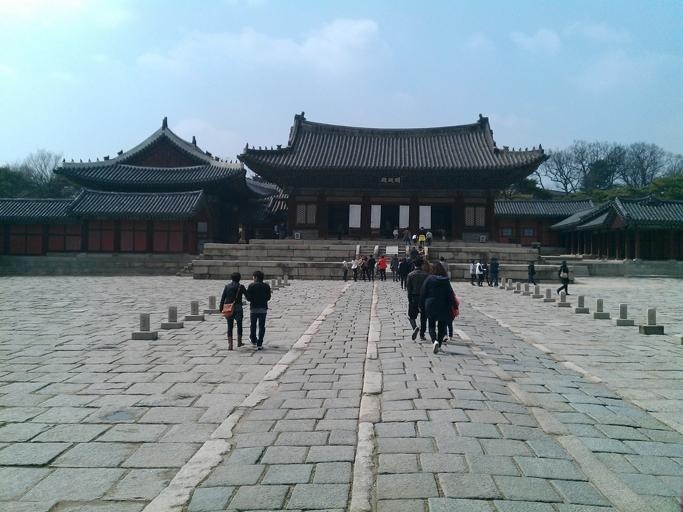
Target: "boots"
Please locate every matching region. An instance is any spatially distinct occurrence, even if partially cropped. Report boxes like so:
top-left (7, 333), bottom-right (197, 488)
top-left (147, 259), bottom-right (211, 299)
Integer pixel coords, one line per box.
top-left (228, 337), bottom-right (233, 350)
top-left (238, 334), bottom-right (245, 347)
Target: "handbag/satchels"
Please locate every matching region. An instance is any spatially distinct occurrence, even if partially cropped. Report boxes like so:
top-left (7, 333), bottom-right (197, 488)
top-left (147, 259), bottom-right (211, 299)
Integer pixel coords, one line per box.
top-left (412, 234), bottom-right (419, 240)
top-left (560, 271), bottom-right (567, 278)
top-left (221, 304), bottom-right (234, 318)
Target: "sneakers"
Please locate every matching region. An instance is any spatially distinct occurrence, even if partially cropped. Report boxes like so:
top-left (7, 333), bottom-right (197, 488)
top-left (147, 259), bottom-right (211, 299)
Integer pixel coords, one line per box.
top-left (433, 340), bottom-right (445, 354)
top-left (258, 345), bottom-right (263, 350)
top-left (443, 335), bottom-right (448, 342)
top-left (420, 336), bottom-right (426, 340)
top-left (557, 289), bottom-right (570, 295)
top-left (471, 282), bottom-right (498, 287)
top-left (249, 338), bottom-right (257, 345)
top-left (412, 327), bottom-right (419, 340)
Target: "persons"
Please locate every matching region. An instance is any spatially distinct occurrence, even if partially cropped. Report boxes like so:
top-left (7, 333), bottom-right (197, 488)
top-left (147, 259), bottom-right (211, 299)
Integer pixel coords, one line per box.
top-left (343, 223), bottom-right (460, 354)
top-left (527, 260), bottom-right (538, 286)
top-left (556, 260), bottom-right (571, 296)
top-left (246, 271), bottom-right (272, 350)
top-left (221, 273), bottom-right (247, 350)
top-left (470, 258), bottom-right (499, 288)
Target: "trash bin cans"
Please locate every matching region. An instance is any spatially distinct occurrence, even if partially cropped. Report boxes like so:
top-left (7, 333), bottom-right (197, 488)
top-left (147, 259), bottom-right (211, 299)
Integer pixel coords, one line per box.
top-left (531, 242), bottom-right (542, 255)
